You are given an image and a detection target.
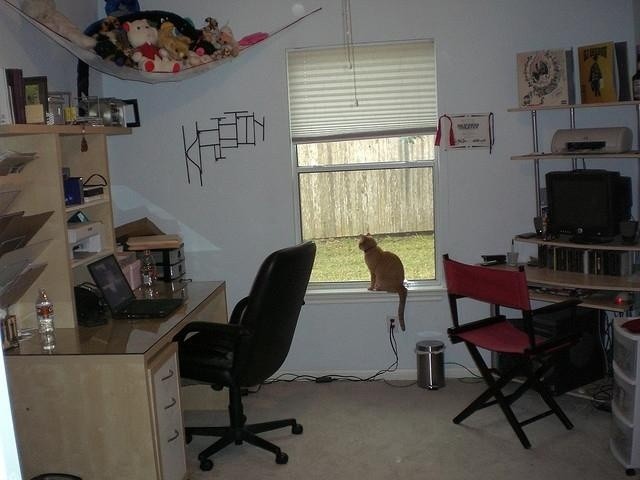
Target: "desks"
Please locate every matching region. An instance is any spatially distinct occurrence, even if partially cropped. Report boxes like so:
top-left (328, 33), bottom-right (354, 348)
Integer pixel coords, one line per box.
top-left (4, 280), bottom-right (228, 480)
top-left (471, 261), bottom-right (640, 405)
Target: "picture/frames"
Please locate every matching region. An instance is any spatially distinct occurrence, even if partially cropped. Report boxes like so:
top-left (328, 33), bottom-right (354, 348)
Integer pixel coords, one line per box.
top-left (24, 76), bottom-right (50, 124)
top-left (122, 99), bottom-right (142, 127)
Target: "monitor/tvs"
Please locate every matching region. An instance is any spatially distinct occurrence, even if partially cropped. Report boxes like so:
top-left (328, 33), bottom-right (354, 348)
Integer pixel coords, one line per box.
top-left (545, 169), bottom-right (632, 244)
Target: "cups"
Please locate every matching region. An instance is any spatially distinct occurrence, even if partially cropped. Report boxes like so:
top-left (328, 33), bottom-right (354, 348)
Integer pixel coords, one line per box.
top-left (507, 252), bottom-right (519, 267)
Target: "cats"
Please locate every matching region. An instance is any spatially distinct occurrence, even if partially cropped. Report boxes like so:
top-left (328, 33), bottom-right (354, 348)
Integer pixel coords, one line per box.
top-left (359, 233), bottom-right (408, 331)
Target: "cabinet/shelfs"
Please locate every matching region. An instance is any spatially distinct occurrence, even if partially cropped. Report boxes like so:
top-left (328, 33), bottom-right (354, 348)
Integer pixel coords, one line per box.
top-left (0, 126), bottom-right (134, 328)
top-left (607, 316), bottom-right (640, 476)
top-left (506, 100), bottom-right (640, 253)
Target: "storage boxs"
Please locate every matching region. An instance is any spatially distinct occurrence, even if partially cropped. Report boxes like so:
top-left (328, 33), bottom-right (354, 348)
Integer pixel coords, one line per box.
top-left (125, 243), bottom-right (186, 283)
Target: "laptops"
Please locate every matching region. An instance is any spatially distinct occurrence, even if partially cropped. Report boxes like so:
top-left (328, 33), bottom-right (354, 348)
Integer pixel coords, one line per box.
top-left (87, 253), bottom-right (185, 319)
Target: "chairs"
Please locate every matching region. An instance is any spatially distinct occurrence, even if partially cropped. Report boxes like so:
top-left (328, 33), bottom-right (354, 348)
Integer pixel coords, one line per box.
top-left (442, 253), bottom-right (583, 449)
top-left (173, 239), bottom-right (318, 471)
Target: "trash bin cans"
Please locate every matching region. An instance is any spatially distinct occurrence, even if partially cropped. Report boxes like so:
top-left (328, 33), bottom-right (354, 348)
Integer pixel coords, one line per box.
top-left (415, 340), bottom-right (446, 390)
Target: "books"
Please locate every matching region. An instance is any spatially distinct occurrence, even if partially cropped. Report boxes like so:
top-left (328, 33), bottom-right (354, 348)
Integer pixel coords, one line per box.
top-left (126, 234), bottom-right (183, 251)
top-left (0, 67), bottom-right (71, 125)
top-left (517, 41), bottom-right (621, 106)
top-left (537, 243), bottom-right (640, 276)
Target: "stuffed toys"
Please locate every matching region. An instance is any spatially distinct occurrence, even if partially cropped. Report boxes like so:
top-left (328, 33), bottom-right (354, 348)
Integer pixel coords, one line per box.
top-left (23, 0), bottom-right (239, 72)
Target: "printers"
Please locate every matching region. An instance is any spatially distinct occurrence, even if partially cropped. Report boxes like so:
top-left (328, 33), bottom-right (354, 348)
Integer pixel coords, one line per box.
top-left (550, 127), bottom-right (633, 154)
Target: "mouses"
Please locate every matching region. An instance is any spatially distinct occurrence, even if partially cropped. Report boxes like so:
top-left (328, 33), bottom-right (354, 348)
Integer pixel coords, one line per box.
top-left (617, 293), bottom-right (633, 302)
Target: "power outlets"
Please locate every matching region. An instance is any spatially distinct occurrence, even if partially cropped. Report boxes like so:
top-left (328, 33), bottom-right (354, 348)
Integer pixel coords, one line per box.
top-left (386, 316), bottom-right (400, 334)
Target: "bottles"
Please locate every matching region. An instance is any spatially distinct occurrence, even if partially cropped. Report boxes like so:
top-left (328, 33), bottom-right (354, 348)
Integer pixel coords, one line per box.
top-left (139, 248), bottom-right (158, 289)
top-left (36, 285), bottom-right (54, 336)
top-left (38, 332), bottom-right (57, 354)
top-left (142, 285), bottom-right (159, 299)
top-left (630, 45), bottom-right (640, 101)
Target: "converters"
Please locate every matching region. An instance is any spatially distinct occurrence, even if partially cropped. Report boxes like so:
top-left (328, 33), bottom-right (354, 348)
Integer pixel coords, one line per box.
top-left (316, 377), bottom-right (332, 383)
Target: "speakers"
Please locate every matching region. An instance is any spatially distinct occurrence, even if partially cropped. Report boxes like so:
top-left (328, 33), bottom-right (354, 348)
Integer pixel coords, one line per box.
top-left (534, 217), bottom-right (550, 237)
top-left (619, 221), bottom-right (638, 245)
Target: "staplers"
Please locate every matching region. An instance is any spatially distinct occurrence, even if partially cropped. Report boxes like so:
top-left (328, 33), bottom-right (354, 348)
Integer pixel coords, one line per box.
top-left (481, 255), bottom-right (507, 266)
